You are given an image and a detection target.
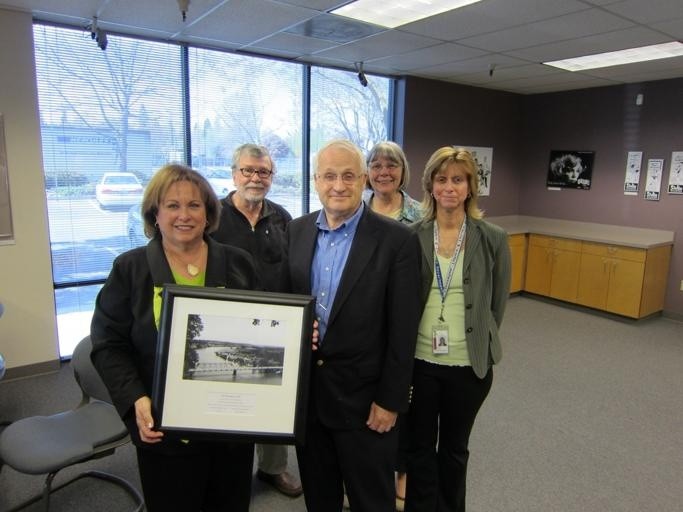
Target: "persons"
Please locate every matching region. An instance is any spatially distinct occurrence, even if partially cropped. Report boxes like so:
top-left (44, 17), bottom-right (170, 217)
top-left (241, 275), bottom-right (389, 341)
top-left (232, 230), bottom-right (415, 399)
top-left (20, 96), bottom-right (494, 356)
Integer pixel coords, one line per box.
top-left (408, 147), bottom-right (513, 511)
top-left (439, 336), bottom-right (448, 349)
top-left (208, 143), bottom-right (304, 496)
top-left (474, 156), bottom-right (489, 188)
top-left (363, 142), bottom-right (427, 510)
top-left (287, 138), bottom-right (423, 511)
top-left (89, 167), bottom-right (260, 511)
top-left (550, 154), bottom-right (590, 188)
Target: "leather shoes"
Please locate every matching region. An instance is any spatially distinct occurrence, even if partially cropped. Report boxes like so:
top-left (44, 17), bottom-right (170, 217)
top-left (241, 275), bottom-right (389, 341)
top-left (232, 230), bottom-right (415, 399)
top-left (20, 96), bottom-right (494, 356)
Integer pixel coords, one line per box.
top-left (256, 467), bottom-right (303, 498)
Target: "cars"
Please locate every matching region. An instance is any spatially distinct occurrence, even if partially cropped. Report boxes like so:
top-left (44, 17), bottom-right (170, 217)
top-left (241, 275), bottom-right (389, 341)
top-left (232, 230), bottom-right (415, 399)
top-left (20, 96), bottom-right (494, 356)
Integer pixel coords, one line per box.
top-left (96, 171), bottom-right (145, 211)
top-left (204, 167), bottom-right (237, 198)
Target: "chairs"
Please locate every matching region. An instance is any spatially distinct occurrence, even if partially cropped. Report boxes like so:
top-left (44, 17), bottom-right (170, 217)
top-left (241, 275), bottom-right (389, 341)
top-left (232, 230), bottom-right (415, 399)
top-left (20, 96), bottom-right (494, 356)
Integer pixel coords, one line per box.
top-left (0, 332), bottom-right (144, 511)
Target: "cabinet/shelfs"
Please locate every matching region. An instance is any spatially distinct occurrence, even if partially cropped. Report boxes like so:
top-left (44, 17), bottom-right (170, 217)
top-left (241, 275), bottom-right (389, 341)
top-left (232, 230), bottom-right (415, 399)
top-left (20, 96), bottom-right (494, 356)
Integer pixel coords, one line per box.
top-left (523, 232), bottom-right (674, 323)
top-left (509, 232), bottom-right (527, 294)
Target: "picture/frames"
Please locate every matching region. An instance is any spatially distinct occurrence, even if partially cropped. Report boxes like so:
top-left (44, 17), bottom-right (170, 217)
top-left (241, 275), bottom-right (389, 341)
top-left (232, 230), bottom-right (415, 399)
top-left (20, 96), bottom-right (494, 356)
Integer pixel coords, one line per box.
top-left (148, 283), bottom-right (318, 445)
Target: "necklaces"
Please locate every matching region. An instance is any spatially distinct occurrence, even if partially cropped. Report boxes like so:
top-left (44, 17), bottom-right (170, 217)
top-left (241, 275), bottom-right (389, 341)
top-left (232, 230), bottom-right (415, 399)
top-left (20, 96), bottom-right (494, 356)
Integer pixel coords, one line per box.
top-left (164, 252), bottom-right (204, 277)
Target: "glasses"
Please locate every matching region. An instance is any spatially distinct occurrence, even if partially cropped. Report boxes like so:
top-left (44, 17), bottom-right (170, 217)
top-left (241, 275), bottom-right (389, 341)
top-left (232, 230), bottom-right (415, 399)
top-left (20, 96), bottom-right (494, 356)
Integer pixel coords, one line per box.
top-left (368, 160), bottom-right (402, 173)
top-left (234, 166), bottom-right (273, 178)
top-left (315, 170), bottom-right (363, 184)
